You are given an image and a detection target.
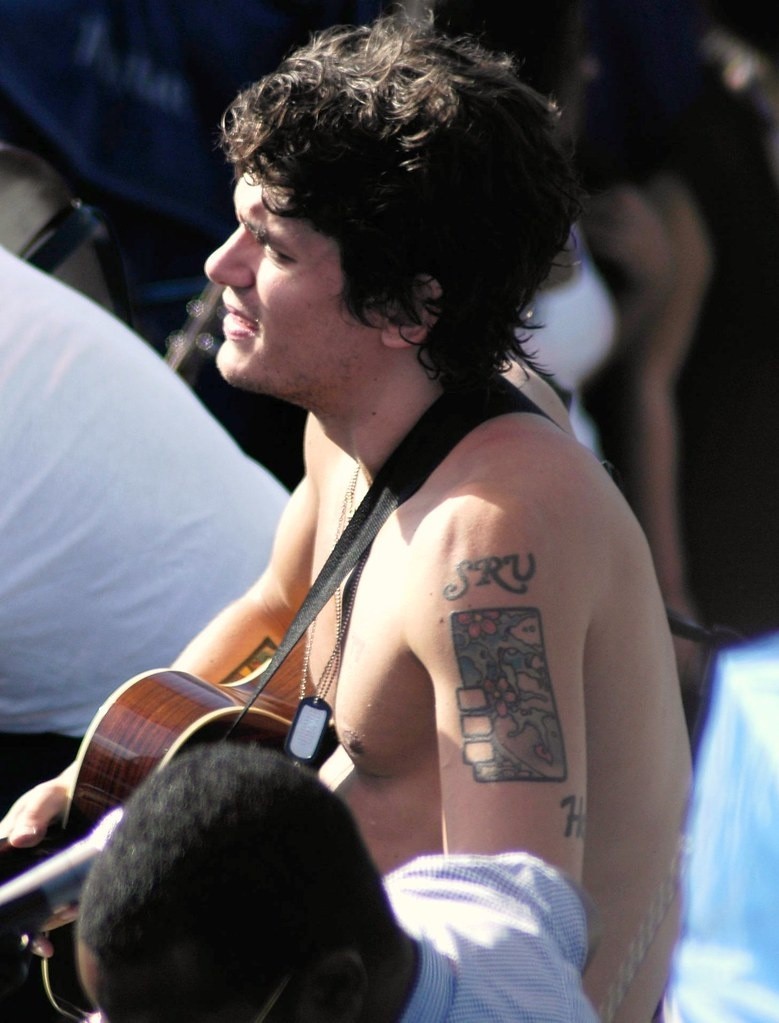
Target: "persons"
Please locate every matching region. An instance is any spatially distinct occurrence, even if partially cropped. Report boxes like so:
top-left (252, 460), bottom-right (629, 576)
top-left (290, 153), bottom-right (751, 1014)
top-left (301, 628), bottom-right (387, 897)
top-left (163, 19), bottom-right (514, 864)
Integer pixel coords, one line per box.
top-left (0, 0), bottom-right (779, 1022)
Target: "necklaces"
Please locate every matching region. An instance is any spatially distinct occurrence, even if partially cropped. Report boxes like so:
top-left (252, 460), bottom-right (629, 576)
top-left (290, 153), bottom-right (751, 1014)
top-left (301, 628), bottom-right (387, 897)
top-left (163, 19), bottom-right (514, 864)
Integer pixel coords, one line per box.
top-left (285, 462), bottom-right (405, 761)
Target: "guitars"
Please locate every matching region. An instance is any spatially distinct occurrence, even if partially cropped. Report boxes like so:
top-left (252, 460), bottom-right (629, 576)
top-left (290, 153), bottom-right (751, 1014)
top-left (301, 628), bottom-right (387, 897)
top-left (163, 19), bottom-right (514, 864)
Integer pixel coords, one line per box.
top-left (1, 663), bottom-right (339, 1004)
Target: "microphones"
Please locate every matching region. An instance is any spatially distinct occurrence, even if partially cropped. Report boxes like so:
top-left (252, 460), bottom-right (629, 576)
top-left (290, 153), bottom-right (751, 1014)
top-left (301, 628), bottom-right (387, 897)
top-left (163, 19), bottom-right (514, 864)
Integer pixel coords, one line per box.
top-left (0, 808), bottom-right (124, 918)
